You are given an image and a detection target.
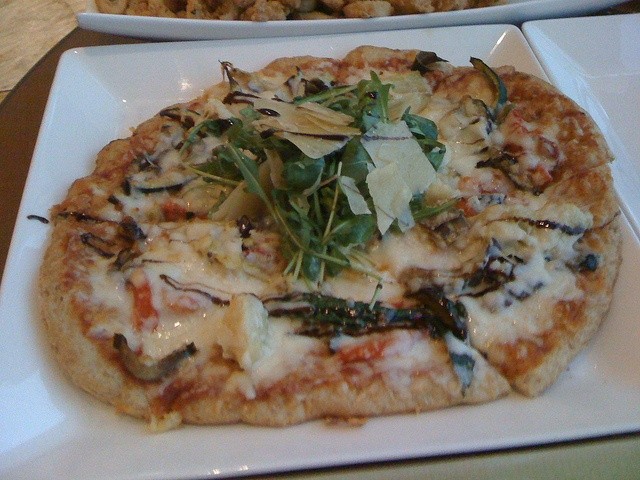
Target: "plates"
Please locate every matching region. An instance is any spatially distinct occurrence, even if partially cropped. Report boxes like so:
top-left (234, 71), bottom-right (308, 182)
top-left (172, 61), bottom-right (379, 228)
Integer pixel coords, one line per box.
top-left (76, 0), bottom-right (628, 40)
top-left (522, 12), bottom-right (640, 239)
top-left (1, 22), bottom-right (639, 476)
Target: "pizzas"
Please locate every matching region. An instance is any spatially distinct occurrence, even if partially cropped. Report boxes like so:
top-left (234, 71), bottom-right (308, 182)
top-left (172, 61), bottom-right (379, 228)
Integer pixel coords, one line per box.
top-left (36, 46), bottom-right (619, 433)
